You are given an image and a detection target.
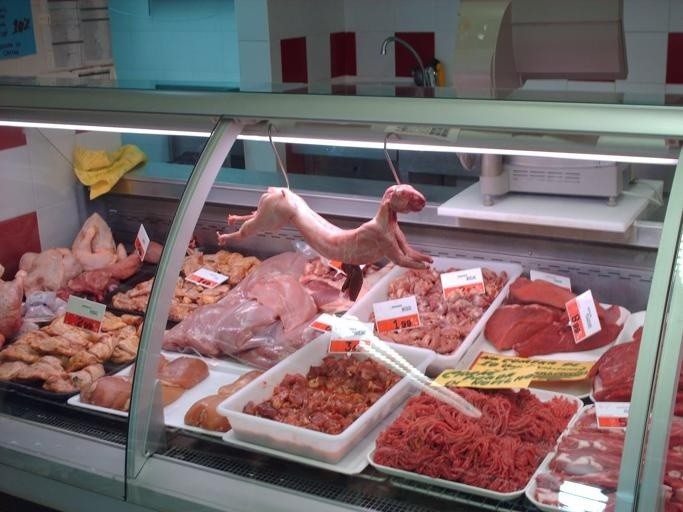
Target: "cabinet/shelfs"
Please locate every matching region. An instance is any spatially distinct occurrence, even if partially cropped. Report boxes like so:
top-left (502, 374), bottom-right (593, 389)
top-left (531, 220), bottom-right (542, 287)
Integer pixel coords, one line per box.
top-left (0, 83), bottom-right (683, 512)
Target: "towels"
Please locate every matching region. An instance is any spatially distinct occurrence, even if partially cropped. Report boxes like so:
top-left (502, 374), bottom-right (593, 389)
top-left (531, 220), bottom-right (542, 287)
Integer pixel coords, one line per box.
top-left (72, 143), bottom-right (146, 200)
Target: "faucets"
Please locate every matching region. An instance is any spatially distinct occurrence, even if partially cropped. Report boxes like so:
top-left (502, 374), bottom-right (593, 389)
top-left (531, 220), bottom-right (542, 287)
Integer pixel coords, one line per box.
top-left (379, 35), bottom-right (440, 88)
